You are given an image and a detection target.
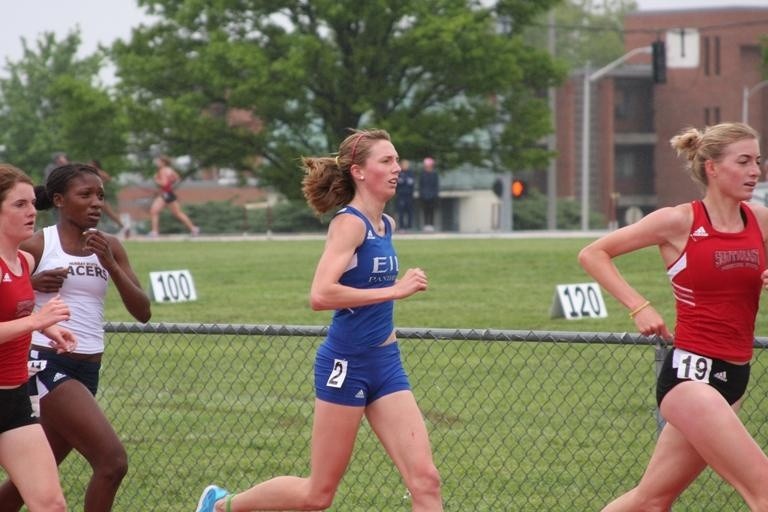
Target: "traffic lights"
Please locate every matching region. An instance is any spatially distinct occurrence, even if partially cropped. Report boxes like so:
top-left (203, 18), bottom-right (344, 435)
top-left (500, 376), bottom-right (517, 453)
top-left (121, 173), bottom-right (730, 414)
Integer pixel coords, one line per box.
top-left (512, 178), bottom-right (529, 201)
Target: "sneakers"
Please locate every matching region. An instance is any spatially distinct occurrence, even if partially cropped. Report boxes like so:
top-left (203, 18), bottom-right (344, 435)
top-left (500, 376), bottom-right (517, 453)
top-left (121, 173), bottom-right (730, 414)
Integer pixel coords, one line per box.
top-left (196, 485), bottom-right (229, 512)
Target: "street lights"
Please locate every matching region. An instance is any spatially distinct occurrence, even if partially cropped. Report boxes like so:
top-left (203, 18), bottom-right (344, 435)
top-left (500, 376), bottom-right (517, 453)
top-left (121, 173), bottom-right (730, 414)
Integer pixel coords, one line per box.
top-left (581, 45), bottom-right (654, 231)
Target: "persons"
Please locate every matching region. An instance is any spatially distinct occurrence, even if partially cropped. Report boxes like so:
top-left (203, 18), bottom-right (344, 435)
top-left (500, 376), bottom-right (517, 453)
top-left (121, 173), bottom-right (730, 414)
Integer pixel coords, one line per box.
top-left (0, 163), bottom-right (150, 512)
top-left (577, 122), bottom-right (768, 512)
top-left (195, 125), bottom-right (445, 512)
top-left (396, 158), bottom-right (415, 233)
top-left (44, 151), bottom-right (69, 223)
top-left (419, 156), bottom-right (438, 234)
top-left (147, 156), bottom-right (200, 238)
top-left (89, 158), bottom-right (131, 238)
top-left (0, 162), bottom-right (77, 512)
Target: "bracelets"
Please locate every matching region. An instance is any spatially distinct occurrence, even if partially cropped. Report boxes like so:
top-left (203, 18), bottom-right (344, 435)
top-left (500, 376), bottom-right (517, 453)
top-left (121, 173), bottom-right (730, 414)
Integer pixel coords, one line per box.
top-left (629, 301), bottom-right (650, 318)
